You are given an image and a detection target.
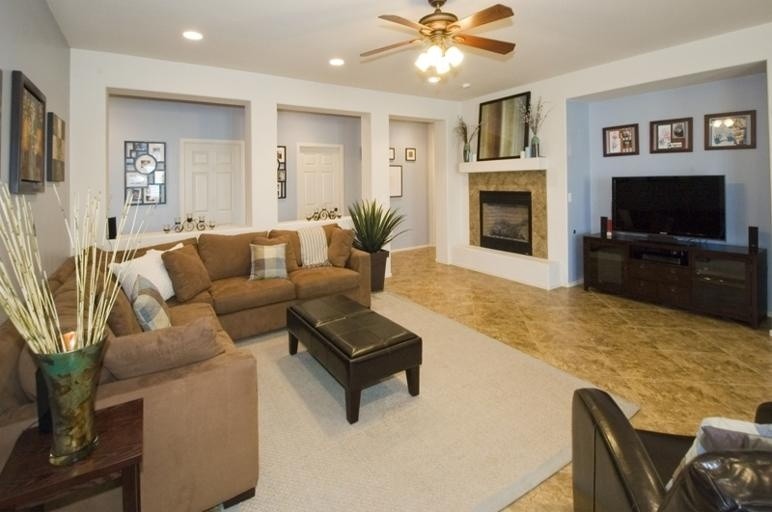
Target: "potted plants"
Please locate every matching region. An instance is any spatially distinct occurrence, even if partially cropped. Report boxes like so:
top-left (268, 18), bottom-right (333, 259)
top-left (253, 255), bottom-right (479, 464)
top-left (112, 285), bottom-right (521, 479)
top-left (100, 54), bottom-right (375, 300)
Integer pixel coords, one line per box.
top-left (344, 196), bottom-right (412, 292)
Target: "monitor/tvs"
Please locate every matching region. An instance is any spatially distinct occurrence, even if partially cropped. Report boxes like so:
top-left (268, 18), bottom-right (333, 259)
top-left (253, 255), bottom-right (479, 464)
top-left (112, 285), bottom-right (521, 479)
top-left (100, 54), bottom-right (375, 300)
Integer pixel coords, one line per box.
top-left (612, 175), bottom-right (726, 242)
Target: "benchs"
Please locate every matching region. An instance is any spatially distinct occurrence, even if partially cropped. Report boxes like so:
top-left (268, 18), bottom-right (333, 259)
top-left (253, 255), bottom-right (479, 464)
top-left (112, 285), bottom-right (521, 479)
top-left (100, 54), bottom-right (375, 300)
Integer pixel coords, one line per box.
top-left (286, 292), bottom-right (422, 425)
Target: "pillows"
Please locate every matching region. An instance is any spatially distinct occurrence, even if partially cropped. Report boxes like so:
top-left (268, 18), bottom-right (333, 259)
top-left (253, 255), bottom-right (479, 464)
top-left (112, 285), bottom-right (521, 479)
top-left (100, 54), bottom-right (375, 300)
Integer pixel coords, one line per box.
top-left (130, 273), bottom-right (172, 332)
top-left (106, 241), bottom-right (184, 303)
top-left (95, 278), bottom-right (143, 337)
top-left (162, 243), bottom-right (212, 303)
top-left (666, 416), bottom-right (772, 492)
top-left (253, 233), bottom-right (299, 272)
top-left (328, 227), bottom-right (353, 268)
top-left (247, 243), bottom-right (290, 280)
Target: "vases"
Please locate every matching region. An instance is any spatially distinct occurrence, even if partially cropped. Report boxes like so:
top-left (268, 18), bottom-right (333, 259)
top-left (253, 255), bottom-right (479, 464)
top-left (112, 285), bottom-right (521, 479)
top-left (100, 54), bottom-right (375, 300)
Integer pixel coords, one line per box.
top-left (531, 134), bottom-right (542, 161)
top-left (29, 327), bottom-right (110, 465)
top-left (463, 143), bottom-right (471, 162)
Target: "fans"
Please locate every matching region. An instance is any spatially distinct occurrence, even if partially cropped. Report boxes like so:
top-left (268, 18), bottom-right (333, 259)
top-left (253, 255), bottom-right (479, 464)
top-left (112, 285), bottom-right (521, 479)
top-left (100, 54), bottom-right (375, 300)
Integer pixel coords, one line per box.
top-left (360, 0), bottom-right (516, 58)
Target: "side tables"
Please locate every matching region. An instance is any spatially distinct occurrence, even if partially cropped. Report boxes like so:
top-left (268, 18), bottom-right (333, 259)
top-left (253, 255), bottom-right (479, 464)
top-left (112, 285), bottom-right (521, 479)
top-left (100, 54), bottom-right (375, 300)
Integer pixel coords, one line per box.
top-left (1, 397), bottom-right (146, 512)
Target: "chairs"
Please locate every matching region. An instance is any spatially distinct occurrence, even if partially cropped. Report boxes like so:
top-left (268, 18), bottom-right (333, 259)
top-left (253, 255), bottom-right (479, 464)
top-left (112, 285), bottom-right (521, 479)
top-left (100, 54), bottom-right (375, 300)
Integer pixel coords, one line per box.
top-left (572, 388), bottom-right (772, 512)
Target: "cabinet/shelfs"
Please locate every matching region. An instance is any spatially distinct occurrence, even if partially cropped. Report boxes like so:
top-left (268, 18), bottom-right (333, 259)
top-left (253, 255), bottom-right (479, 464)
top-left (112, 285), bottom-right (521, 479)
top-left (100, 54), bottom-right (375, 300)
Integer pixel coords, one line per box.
top-left (582, 232), bottom-right (766, 328)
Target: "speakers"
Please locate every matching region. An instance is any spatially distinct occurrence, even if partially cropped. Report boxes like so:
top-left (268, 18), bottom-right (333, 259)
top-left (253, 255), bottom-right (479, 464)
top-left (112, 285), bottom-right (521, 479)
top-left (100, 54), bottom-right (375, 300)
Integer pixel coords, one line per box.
top-left (109, 217), bottom-right (116, 239)
top-left (748, 226), bottom-right (758, 252)
top-left (600, 217), bottom-right (608, 235)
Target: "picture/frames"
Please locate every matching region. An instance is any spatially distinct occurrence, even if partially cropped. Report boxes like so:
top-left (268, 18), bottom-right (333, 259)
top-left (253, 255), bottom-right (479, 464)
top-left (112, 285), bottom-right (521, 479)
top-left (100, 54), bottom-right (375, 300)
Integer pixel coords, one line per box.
top-left (602, 123), bottom-right (639, 156)
top-left (389, 148), bottom-right (395, 160)
top-left (703, 110), bottom-right (756, 149)
top-left (477, 91), bottom-right (530, 160)
top-left (405, 148), bottom-right (416, 160)
top-left (11, 70), bottom-right (46, 194)
top-left (650, 117), bottom-right (694, 152)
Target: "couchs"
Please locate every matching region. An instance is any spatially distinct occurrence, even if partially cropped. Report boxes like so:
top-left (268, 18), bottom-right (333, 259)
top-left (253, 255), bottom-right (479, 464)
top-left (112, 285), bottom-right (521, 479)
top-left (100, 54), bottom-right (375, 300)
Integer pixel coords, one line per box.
top-left (0, 223), bottom-right (372, 512)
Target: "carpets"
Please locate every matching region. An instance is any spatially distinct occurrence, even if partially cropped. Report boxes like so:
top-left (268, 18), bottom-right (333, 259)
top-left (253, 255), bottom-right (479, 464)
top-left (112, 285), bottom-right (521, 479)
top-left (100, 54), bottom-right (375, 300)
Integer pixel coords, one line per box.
top-left (229, 290), bottom-right (641, 512)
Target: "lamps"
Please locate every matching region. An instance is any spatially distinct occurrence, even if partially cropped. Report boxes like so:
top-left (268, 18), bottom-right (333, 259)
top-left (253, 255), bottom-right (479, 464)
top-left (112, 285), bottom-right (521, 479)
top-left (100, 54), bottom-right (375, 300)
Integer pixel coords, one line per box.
top-left (415, 37), bottom-right (465, 86)
